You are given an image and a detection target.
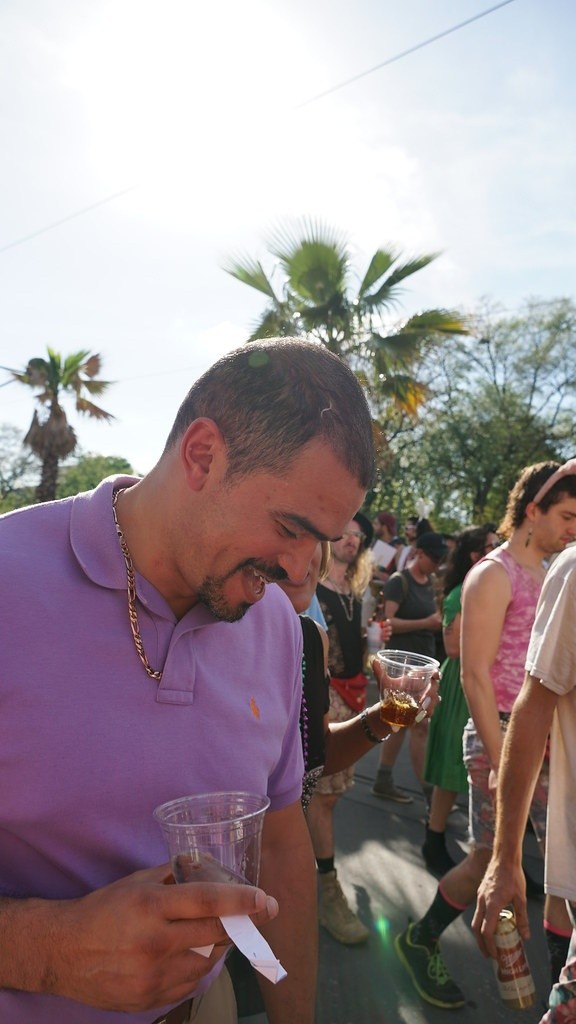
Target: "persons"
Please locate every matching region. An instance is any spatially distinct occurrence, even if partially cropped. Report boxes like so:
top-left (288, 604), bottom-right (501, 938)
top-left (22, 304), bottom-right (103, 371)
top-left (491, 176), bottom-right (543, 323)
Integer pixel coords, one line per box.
top-left (300, 593), bottom-right (370, 945)
top-left (372, 454), bottom-right (576, 1024)
top-left (300, 615), bottom-right (442, 816)
top-left (315, 511), bottom-right (375, 795)
top-left (0, 338), bottom-right (372, 1024)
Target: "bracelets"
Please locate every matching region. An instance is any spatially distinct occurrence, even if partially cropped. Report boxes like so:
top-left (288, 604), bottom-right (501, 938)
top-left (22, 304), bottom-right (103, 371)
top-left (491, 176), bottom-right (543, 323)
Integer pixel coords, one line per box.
top-left (361, 707), bottom-right (390, 743)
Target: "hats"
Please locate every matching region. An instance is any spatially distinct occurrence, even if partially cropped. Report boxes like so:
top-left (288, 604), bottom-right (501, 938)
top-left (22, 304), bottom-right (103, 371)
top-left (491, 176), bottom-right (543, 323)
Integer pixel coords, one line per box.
top-left (351, 513), bottom-right (374, 548)
top-left (416, 534), bottom-right (451, 559)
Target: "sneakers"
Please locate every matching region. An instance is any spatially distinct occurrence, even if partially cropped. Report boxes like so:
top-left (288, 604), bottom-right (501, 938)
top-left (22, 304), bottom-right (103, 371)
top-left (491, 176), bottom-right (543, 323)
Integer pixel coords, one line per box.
top-left (315, 870), bottom-right (368, 944)
top-left (393, 923), bottom-right (466, 1008)
top-left (369, 783), bottom-right (414, 803)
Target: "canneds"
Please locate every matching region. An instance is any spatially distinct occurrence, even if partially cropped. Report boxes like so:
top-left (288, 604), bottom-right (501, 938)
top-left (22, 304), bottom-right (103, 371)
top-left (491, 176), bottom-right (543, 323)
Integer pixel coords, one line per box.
top-left (491, 909), bottom-right (536, 1007)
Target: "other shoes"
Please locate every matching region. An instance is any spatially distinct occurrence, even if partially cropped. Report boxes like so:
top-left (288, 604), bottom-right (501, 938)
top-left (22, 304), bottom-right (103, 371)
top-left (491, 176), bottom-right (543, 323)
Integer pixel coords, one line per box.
top-left (419, 841), bottom-right (456, 876)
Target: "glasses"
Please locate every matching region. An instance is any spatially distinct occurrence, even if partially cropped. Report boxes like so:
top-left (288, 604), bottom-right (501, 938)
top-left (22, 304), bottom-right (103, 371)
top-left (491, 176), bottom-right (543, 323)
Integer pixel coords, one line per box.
top-left (342, 531), bottom-right (366, 540)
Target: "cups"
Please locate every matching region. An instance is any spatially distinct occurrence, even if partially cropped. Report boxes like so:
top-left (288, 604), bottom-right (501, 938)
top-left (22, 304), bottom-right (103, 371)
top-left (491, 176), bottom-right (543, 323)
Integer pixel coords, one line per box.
top-left (377, 649), bottom-right (440, 727)
top-left (153, 791), bottom-right (269, 903)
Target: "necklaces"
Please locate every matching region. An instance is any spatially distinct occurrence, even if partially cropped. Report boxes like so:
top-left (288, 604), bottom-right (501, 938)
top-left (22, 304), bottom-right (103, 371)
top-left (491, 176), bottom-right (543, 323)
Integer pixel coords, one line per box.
top-left (111, 488), bottom-right (163, 680)
top-left (327, 576), bottom-right (355, 621)
top-left (300, 654), bottom-right (308, 767)
